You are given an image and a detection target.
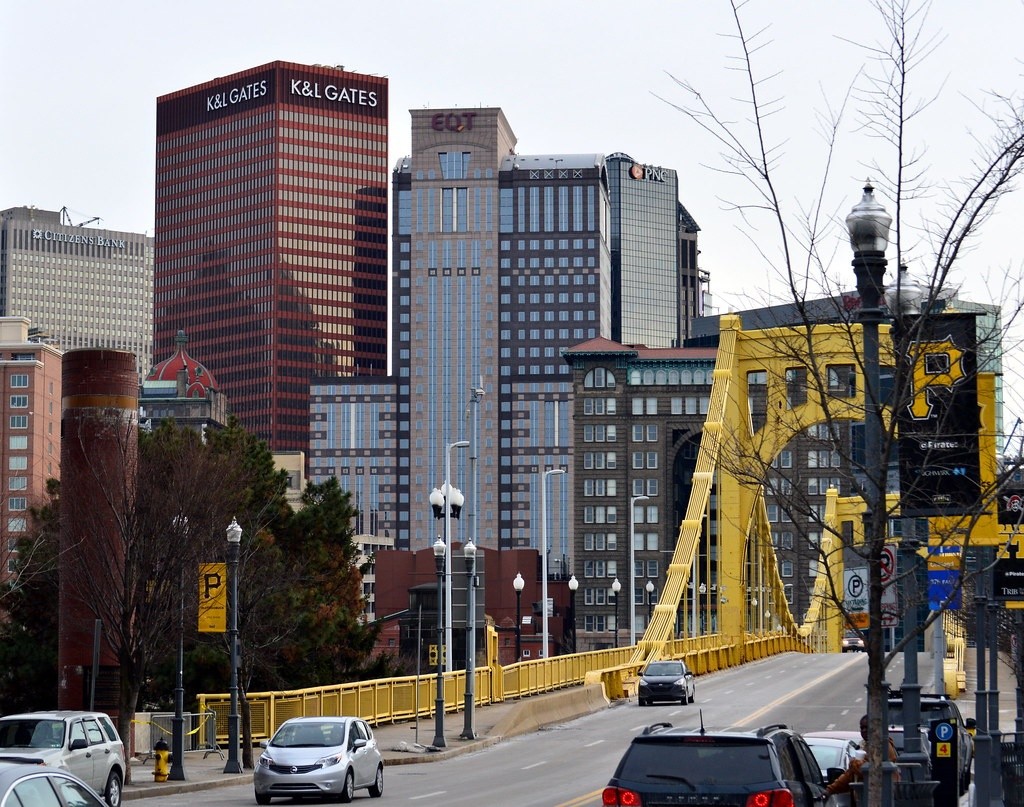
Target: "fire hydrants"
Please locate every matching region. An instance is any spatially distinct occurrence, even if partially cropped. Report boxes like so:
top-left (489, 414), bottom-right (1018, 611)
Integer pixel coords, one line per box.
top-left (151, 737), bottom-right (173, 783)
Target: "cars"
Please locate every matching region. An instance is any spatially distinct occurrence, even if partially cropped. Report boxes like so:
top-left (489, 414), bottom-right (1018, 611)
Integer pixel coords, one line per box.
top-left (253, 716), bottom-right (384, 805)
top-left (841, 630), bottom-right (866, 653)
top-left (0, 710), bottom-right (127, 807)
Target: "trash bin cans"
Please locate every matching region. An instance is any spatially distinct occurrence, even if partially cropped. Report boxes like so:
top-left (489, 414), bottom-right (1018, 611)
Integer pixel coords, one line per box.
top-left (850, 763), bottom-right (940, 807)
top-left (1000, 732), bottom-right (1024, 807)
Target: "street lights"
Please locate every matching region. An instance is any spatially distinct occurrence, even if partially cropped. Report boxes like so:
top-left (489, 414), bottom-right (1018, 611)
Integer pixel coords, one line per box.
top-left (512, 571), bottom-right (526, 663)
top-left (645, 579), bottom-right (655, 623)
top-left (843, 175), bottom-right (896, 807)
top-left (541, 469), bottom-right (565, 658)
top-left (432, 533), bottom-right (447, 747)
top-left (568, 573), bottom-right (579, 654)
top-left (167, 506), bottom-right (187, 780)
top-left (224, 515), bottom-right (243, 773)
top-left (888, 262), bottom-right (941, 807)
top-left (700, 582), bottom-right (706, 636)
top-left (460, 537), bottom-right (477, 739)
top-left (630, 496), bottom-right (650, 646)
top-left (445, 440), bottom-right (469, 672)
top-left (611, 577), bottom-right (622, 649)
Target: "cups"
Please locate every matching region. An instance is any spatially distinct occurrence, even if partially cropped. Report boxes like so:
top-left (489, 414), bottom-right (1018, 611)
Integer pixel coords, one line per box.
top-left (856, 750), bottom-right (866, 760)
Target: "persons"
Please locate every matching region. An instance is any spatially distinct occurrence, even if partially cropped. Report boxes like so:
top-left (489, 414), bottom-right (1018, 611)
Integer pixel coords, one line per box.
top-left (858, 714), bottom-right (897, 762)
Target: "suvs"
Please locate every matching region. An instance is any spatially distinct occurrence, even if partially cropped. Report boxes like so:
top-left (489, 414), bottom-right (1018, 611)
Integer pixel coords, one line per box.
top-left (601, 693), bottom-right (976, 807)
top-left (637, 659), bottom-right (696, 706)
